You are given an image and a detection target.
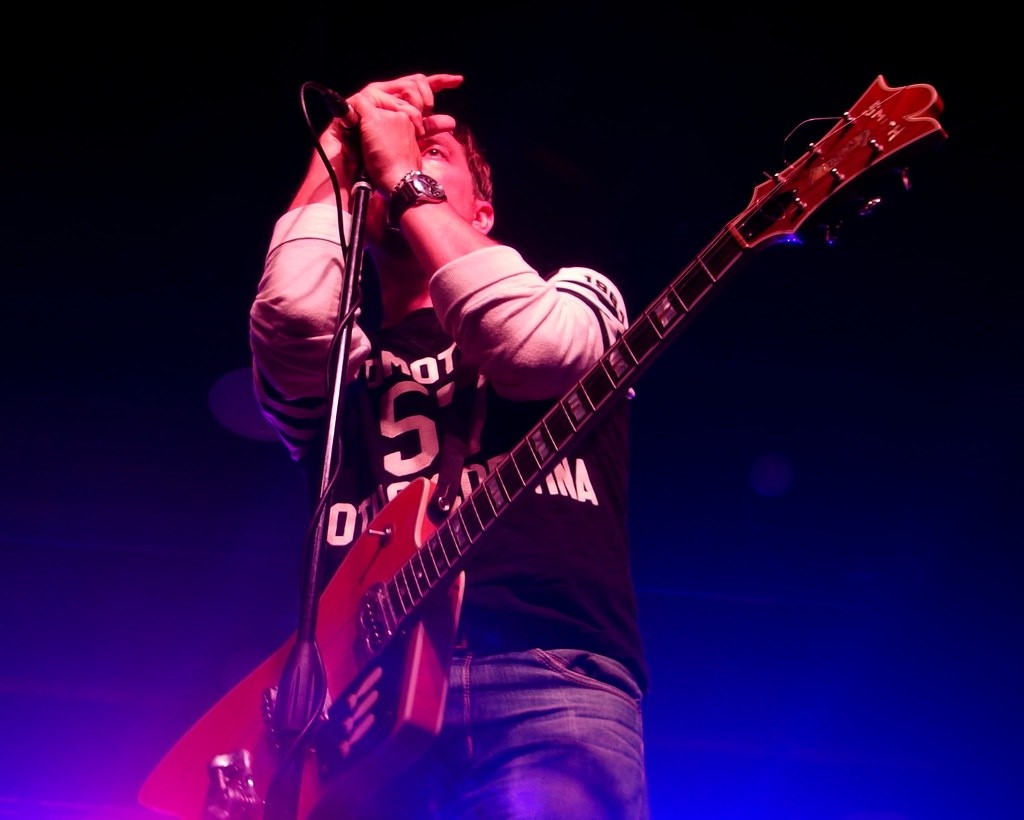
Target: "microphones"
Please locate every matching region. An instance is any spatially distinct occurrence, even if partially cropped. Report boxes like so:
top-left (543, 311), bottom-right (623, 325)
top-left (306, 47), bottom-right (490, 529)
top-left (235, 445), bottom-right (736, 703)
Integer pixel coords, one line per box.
top-left (321, 86), bottom-right (363, 162)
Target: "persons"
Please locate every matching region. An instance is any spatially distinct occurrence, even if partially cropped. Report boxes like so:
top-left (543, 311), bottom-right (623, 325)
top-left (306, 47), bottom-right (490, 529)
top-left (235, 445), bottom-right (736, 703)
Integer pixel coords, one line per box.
top-left (137, 72), bottom-right (654, 819)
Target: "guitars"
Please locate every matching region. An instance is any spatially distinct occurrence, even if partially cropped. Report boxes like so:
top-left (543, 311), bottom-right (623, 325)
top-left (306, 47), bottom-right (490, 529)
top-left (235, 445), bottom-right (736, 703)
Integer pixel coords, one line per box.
top-left (134, 75), bottom-right (949, 819)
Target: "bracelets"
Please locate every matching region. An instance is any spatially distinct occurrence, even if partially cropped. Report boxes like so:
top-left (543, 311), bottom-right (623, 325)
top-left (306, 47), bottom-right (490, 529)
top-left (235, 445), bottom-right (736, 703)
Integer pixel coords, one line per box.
top-left (388, 169), bottom-right (422, 223)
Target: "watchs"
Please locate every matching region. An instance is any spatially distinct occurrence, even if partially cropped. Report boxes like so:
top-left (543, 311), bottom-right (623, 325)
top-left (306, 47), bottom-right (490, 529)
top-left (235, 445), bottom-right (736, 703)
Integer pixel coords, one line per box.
top-left (386, 173), bottom-right (449, 231)
